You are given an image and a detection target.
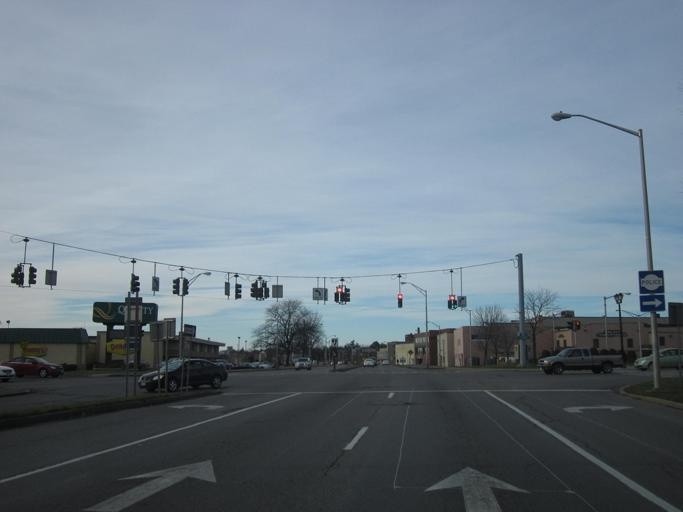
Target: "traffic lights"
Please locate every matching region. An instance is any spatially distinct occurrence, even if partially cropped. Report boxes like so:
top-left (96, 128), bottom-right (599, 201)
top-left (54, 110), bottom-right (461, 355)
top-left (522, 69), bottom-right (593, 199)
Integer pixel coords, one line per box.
top-left (343, 286), bottom-right (350, 303)
top-left (171, 278), bottom-right (180, 296)
top-left (397, 294), bottom-right (402, 308)
top-left (447, 295), bottom-right (457, 311)
top-left (335, 285), bottom-right (343, 304)
top-left (11, 266), bottom-right (18, 284)
top-left (234, 284), bottom-right (241, 300)
top-left (181, 278), bottom-right (188, 296)
top-left (574, 319), bottom-right (580, 330)
top-left (130, 273), bottom-right (140, 293)
top-left (28, 266), bottom-right (37, 285)
top-left (251, 280), bottom-right (259, 301)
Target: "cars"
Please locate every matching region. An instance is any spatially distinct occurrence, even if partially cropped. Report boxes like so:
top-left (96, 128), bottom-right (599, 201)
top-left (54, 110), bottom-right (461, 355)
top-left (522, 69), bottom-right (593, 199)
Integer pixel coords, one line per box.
top-left (632, 347), bottom-right (682, 372)
top-left (214, 357), bottom-right (389, 371)
top-left (0, 355), bottom-right (64, 382)
top-left (137, 358), bottom-right (227, 394)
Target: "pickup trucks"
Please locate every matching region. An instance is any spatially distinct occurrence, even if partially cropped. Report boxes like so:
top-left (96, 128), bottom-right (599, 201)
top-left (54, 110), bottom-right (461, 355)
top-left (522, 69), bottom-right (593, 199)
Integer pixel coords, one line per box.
top-left (538, 347), bottom-right (623, 375)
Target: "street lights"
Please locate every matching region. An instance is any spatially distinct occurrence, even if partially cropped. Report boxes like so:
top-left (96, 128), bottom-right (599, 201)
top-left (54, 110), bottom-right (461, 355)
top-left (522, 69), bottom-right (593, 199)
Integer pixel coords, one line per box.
top-left (551, 110), bottom-right (662, 390)
top-left (615, 308), bottom-right (650, 358)
top-left (179, 271), bottom-right (212, 361)
top-left (6, 320), bottom-right (10, 328)
top-left (603, 292), bottom-right (631, 346)
top-left (324, 334), bottom-right (337, 363)
top-left (427, 321), bottom-right (441, 367)
